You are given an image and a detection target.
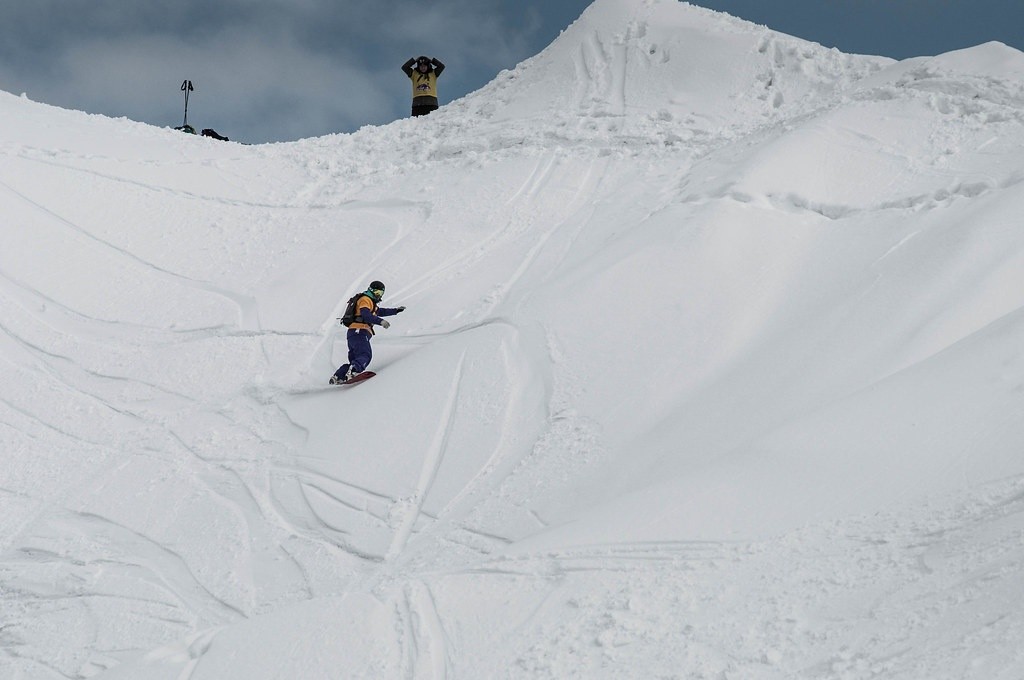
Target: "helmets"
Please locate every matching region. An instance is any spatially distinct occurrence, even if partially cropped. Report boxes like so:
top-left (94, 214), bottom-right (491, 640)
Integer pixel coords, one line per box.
top-left (368, 281), bottom-right (385, 297)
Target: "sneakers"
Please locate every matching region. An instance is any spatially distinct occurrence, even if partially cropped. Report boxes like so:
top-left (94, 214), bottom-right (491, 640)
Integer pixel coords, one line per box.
top-left (329, 377), bottom-right (345, 385)
top-left (345, 372), bottom-right (361, 381)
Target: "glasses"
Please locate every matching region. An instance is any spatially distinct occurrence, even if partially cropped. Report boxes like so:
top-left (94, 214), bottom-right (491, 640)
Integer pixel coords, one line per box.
top-left (372, 289), bottom-right (384, 297)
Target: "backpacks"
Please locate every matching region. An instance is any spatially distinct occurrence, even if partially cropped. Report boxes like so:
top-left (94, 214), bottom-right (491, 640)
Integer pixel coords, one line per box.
top-left (340, 293), bottom-right (375, 327)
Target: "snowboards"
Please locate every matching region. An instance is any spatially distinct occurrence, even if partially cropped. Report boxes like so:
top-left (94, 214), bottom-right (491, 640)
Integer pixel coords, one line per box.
top-left (335, 370), bottom-right (375, 384)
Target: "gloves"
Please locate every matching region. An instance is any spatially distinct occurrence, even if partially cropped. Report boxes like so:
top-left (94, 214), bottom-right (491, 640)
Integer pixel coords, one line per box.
top-left (398, 306), bottom-right (406, 313)
top-left (380, 319), bottom-right (390, 329)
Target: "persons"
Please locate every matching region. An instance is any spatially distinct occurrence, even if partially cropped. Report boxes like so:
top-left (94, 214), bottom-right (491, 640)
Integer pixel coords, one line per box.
top-left (328, 280), bottom-right (407, 384)
top-left (400, 55), bottom-right (445, 116)
